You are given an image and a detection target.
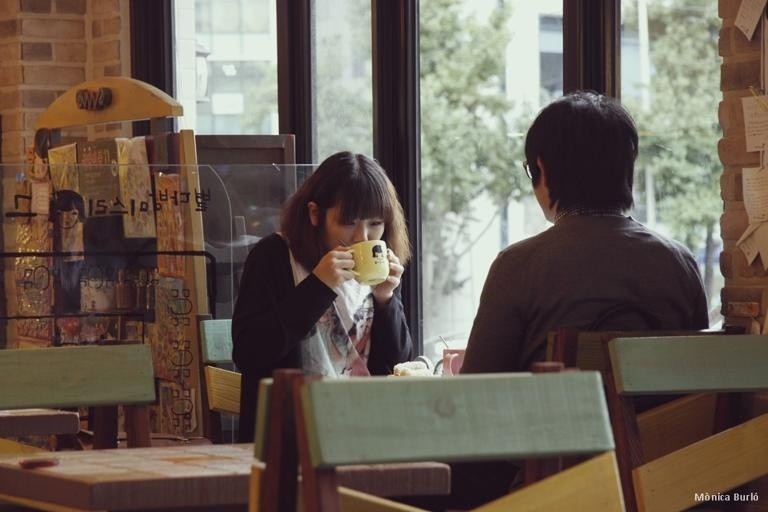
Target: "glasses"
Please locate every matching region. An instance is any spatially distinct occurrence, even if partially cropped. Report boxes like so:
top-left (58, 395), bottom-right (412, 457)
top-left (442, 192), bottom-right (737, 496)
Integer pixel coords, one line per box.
top-left (523, 159), bottom-right (537, 180)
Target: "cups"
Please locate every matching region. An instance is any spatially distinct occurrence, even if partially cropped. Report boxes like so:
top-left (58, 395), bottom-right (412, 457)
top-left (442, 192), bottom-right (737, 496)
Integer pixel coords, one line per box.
top-left (442, 348), bottom-right (466, 375)
top-left (344, 239), bottom-right (390, 286)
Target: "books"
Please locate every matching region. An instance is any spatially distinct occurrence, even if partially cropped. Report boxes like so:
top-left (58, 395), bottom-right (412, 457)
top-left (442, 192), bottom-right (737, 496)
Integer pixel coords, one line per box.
top-left (17, 136), bottom-right (196, 436)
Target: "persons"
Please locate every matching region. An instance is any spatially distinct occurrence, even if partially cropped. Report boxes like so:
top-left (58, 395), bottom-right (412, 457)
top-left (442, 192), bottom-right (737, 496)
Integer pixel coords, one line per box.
top-left (231, 150), bottom-right (417, 443)
top-left (419, 90), bottom-right (710, 511)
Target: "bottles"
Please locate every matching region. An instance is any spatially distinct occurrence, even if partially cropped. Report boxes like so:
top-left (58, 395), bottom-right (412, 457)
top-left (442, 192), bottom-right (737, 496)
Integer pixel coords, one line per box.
top-left (115, 266), bottom-right (159, 310)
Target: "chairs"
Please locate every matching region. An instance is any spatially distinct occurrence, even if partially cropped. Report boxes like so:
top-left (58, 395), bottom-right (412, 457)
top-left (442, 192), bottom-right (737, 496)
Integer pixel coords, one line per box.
top-left (1, 334), bottom-right (768, 512)
